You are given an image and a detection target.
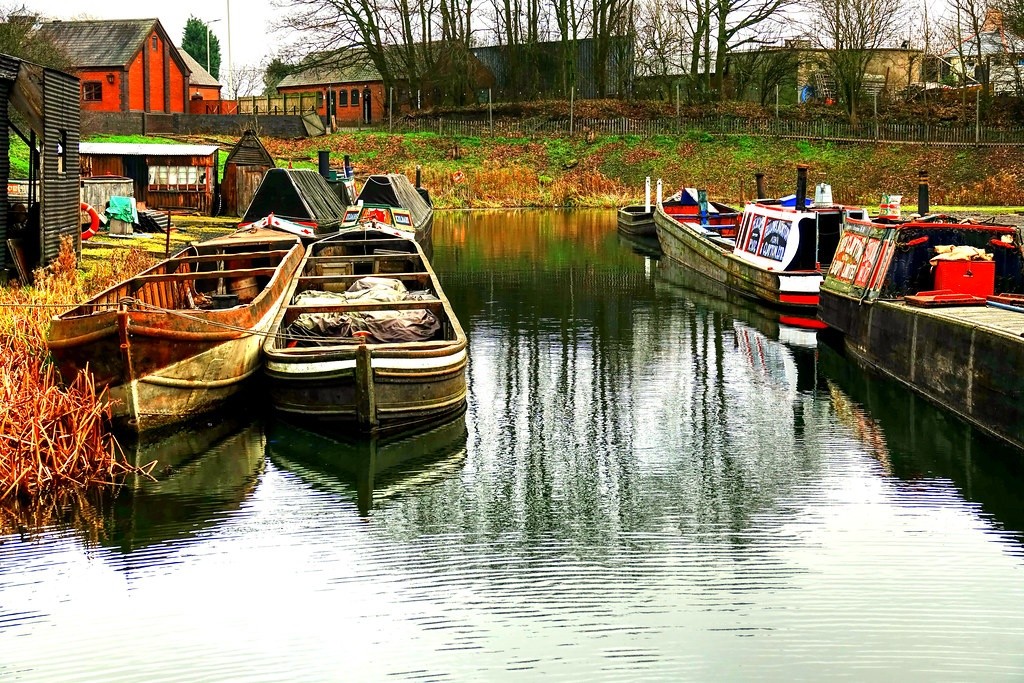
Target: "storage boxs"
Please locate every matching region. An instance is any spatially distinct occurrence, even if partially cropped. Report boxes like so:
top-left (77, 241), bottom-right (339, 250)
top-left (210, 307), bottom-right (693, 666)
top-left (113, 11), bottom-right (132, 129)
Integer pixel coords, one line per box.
top-left (935, 261), bottom-right (996, 300)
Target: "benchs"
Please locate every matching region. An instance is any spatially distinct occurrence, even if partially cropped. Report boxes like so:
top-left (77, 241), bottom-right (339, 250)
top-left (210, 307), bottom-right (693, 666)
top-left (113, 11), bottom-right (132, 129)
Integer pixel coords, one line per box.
top-left (307, 253), bottom-right (421, 265)
top-left (702, 224), bottom-right (735, 230)
top-left (133, 267), bottom-right (277, 291)
top-left (284, 299), bottom-right (448, 328)
top-left (170, 250), bottom-right (289, 264)
top-left (295, 272), bottom-right (430, 290)
top-left (321, 238), bottom-right (409, 245)
top-left (669, 212), bottom-right (739, 219)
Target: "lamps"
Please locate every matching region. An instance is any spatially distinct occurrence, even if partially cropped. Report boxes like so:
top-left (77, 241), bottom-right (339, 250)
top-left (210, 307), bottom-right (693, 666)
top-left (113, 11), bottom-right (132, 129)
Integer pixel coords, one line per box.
top-left (106, 73), bottom-right (114, 84)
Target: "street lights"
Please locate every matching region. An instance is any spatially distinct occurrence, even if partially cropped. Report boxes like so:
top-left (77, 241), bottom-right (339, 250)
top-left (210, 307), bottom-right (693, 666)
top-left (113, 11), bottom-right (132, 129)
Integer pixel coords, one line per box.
top-left (202, 18), bottom-right (224, 73)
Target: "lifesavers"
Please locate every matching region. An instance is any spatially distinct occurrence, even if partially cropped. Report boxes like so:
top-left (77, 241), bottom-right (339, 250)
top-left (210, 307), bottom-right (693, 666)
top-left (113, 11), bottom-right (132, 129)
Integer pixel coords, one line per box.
top-left (80, 203), bottom-right (99, 242)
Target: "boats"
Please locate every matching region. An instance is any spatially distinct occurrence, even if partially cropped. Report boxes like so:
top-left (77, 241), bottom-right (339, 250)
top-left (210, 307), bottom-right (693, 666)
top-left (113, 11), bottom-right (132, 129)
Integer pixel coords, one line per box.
top-left (814, 170), bottom-right (1024, 458)
top-left (235, 150), bottom-right (358, 237)
top-left (337, 165), bottom-right (435, 263)
top-left (262, 221), bottom-right (469, 445)
top-left (616, 177), bottom-right (871, 331)
top-left (44, 225), bottom-right (306, 455)
